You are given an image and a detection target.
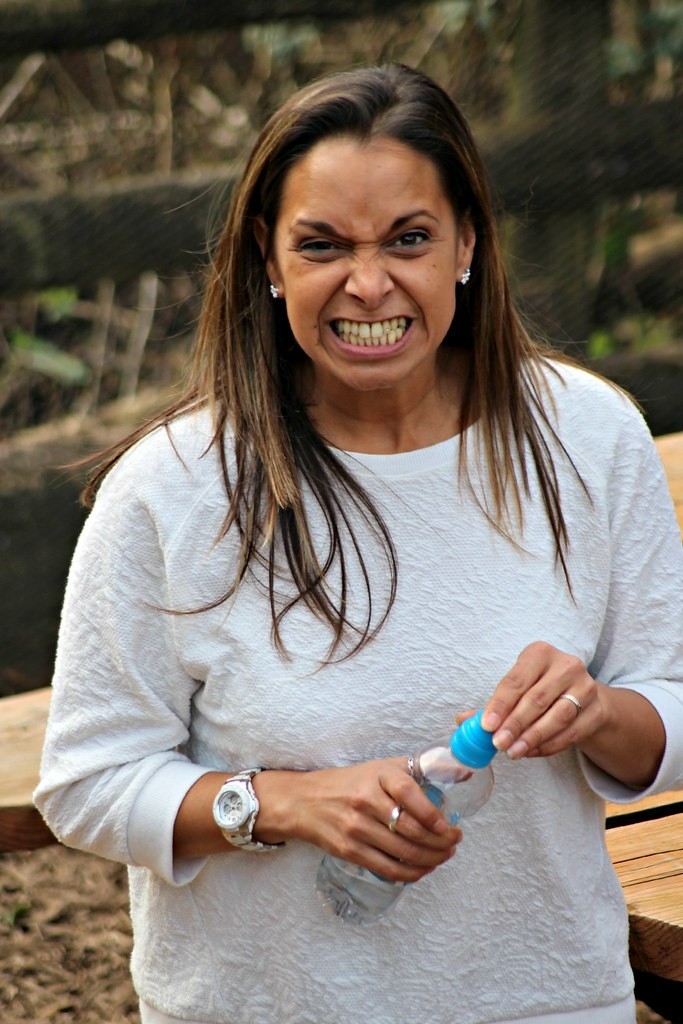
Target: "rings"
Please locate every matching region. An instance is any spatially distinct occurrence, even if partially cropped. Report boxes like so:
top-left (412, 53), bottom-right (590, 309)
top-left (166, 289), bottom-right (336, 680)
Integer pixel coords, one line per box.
top-left (408, 758), bottom-right (414, 778)
top-left (560, 694), bottom-right (581, 717)
top-left (389, 805), bottom-right (402, 833)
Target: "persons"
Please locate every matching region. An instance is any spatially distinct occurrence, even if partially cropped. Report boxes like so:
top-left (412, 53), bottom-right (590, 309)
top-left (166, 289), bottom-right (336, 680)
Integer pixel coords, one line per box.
top-left (32, 61), bottom-right (683, 1024)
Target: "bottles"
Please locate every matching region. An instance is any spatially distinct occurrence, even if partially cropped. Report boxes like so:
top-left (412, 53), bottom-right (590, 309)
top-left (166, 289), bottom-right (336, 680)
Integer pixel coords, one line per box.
top-left (315, 709), bottom-right (500, 925)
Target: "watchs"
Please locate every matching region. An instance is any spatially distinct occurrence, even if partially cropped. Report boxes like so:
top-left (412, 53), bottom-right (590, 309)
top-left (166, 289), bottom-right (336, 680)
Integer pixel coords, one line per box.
top-left (213, 768), bottom-right (285, 853)
